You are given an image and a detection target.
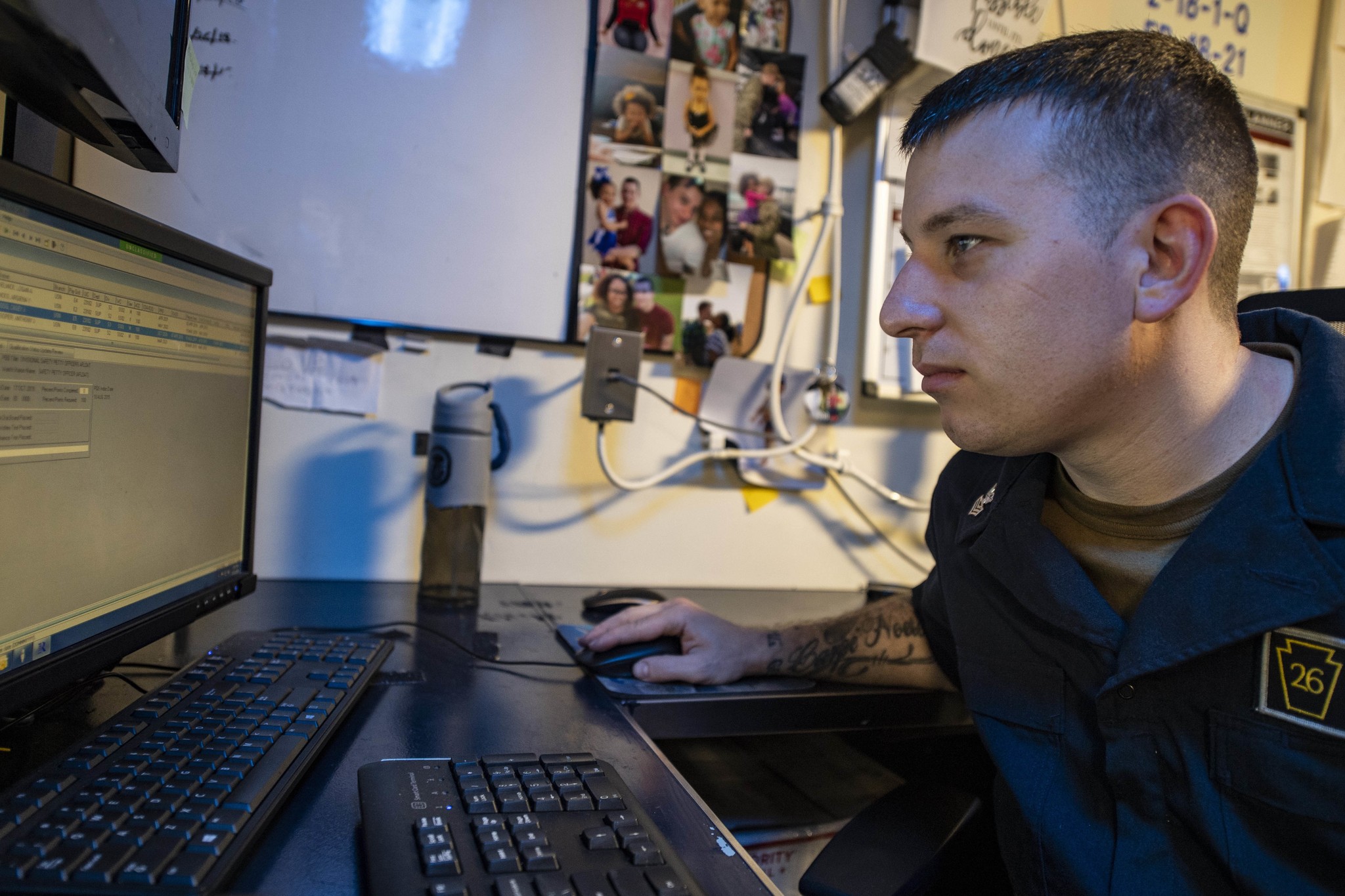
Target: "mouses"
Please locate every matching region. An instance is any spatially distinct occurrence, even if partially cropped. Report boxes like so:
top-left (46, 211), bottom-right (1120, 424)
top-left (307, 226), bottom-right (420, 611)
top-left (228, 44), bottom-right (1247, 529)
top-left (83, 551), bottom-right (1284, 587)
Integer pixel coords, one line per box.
top-left (575, 634), bottom-right (682, 679)
top-left (584, 588), bottom-right (664, 619)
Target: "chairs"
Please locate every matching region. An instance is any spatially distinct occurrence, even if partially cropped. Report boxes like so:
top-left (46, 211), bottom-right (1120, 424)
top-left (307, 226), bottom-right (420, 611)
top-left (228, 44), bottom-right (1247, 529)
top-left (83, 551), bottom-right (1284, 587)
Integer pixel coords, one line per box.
top-left (799, 742), bottom-right (1010, 896)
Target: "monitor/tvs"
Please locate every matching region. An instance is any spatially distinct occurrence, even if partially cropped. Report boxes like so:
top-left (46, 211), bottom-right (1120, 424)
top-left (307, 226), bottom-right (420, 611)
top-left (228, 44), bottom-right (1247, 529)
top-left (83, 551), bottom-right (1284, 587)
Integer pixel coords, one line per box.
top-left (0, 0), bottom-right (193, 174)
top-left (1, 158), bottom-right (274, 790)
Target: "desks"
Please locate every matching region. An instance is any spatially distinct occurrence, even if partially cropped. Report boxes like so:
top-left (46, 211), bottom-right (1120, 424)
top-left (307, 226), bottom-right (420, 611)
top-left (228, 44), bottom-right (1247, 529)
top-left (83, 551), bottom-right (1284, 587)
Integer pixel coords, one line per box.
top-left (0, 580), bottom-right (993, 896)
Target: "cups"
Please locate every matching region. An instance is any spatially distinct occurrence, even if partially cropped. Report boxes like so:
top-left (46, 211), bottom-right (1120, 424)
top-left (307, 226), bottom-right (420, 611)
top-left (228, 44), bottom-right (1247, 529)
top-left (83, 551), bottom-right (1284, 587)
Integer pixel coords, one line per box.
top-left (420, 378), bottom-right (493, 613)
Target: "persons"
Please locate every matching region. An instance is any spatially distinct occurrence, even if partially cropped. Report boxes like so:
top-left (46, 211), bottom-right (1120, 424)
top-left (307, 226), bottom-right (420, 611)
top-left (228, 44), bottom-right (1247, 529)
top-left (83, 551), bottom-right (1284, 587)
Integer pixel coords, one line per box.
top-left (581, 0), bottom-right (796, 369)
top-left (576, 25), bottom-right (1345, 895)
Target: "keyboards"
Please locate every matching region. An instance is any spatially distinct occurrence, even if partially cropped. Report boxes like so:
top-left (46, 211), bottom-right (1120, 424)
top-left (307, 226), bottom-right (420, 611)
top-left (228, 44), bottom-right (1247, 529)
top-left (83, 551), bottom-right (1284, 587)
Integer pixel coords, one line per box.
top-left (0, 629), bottom-right (393, 895)
top-left (356, 752), bottom-right (701, 896)
